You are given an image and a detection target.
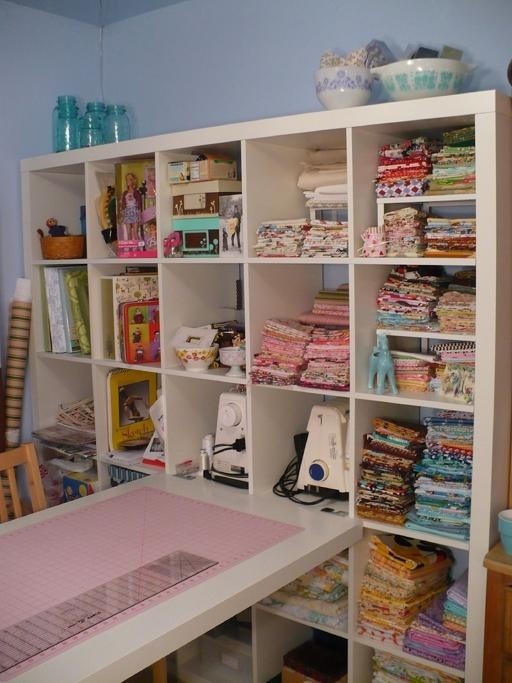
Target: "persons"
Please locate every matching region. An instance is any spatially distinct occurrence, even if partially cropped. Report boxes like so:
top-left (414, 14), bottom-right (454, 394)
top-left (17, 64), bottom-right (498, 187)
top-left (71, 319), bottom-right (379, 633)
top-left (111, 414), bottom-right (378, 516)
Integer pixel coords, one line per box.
top-left (120, 173), bottom-right (143, 252)
top-left (45, 217), bottom-right (71, 238)
top-left (231, 204), bottom-right (242, 248)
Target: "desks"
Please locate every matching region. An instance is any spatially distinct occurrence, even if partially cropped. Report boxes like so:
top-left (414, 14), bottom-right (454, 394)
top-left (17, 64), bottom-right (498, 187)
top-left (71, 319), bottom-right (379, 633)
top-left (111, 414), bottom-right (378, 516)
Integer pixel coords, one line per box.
top-left (1, 465), bottom-right (364, 682)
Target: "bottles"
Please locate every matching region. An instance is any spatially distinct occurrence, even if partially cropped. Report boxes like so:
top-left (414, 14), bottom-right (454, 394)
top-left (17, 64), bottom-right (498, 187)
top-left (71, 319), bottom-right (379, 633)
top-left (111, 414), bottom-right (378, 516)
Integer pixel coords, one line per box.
top-left (51, 93), bottom-right (132, 152)
top-left (198, 449), bottom-right (210, 479)
top-left (202, 433), bottom-right (214, 471)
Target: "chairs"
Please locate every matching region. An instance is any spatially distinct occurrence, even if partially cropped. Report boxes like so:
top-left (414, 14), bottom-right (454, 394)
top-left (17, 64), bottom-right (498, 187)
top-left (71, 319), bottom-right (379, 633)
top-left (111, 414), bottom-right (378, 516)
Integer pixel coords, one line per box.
top-left (1, 440), bottom-right (170, 681)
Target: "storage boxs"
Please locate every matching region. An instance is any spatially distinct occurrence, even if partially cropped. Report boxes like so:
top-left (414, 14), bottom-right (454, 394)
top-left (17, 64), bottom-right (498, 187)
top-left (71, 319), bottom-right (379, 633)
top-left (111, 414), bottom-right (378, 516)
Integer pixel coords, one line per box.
top-left (276, 633), bottom-right (349, 683)
top-left (198, 629), bottom-right (253, 681)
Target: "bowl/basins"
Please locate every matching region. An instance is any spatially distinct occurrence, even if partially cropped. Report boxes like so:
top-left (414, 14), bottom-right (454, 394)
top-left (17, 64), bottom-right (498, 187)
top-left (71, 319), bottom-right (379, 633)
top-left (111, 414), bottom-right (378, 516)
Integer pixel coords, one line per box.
top-left (314, 64), bottom-right (377, 111)
top-left (175, 343), bottom-right (219, 373)
top-left (368, 57), bottom-right (479, 104)
top-left (496, 509), bottom-right (512, 556)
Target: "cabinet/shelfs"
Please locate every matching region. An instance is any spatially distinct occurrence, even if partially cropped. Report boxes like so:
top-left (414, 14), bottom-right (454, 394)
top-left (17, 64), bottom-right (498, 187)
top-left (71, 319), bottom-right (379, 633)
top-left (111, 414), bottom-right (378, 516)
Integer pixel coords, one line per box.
top-left (475, 541), bottom-right (511, 681)
top-left (16, 83), bottom-right (511, 681)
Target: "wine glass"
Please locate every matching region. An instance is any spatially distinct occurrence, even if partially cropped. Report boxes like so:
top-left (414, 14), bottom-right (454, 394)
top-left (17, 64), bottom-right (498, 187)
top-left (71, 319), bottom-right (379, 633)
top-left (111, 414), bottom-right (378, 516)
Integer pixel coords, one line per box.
top-left (218, 345), bottom-right (248, 378)
